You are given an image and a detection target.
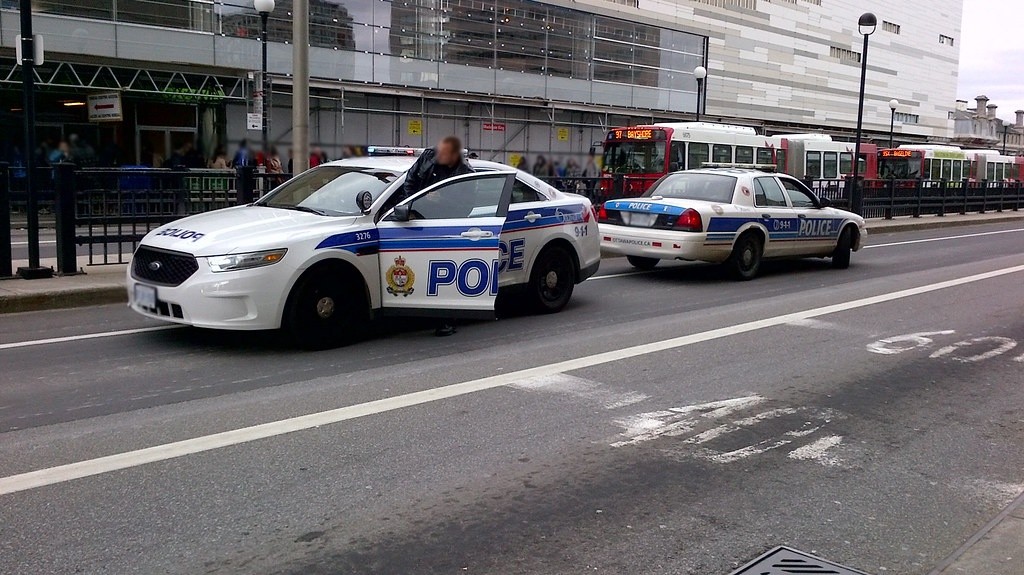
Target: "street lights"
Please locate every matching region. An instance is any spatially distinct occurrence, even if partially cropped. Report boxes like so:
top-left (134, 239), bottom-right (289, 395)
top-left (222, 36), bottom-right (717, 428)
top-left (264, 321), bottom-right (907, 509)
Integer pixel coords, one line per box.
top-left (888, 99), bottom-right (898, 148)
top-left (1002, 119), bottom-right (1011, 156)
top-left (692, 65), bottom-right (706, 122)
top-left (852, 12), bottom-right (877, 216)
top-left (254, 0), bottom-right (275, 165)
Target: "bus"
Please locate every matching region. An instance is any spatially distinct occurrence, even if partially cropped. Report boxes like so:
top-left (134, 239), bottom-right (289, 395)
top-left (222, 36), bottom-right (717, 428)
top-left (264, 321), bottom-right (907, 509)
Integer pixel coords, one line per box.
top-left (591, 122), bottom-right (877, 202)
top-left (876, 143), bottom-right (1024, 193)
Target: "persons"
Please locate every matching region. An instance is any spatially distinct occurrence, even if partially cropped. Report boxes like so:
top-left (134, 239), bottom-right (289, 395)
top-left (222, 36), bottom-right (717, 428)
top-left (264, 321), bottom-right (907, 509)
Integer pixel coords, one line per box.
top-left (404, 136), bottom-right (479, 337)
top-left (468, 148), bottom-right (603, 205)
top-left (1, 132), bottom-right (368, 215)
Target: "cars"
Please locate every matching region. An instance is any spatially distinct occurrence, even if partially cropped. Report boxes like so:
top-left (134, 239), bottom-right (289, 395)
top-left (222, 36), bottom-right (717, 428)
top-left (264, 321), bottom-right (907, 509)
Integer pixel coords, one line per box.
top-left (599, 163), bottom-right (868, 282)
top-left (124, 147), bottom-right (604, 351)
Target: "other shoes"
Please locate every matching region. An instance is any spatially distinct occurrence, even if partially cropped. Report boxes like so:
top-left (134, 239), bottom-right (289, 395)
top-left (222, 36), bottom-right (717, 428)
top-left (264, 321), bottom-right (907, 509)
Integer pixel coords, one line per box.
top-left (434, 322), bottom-right (459, 337)
